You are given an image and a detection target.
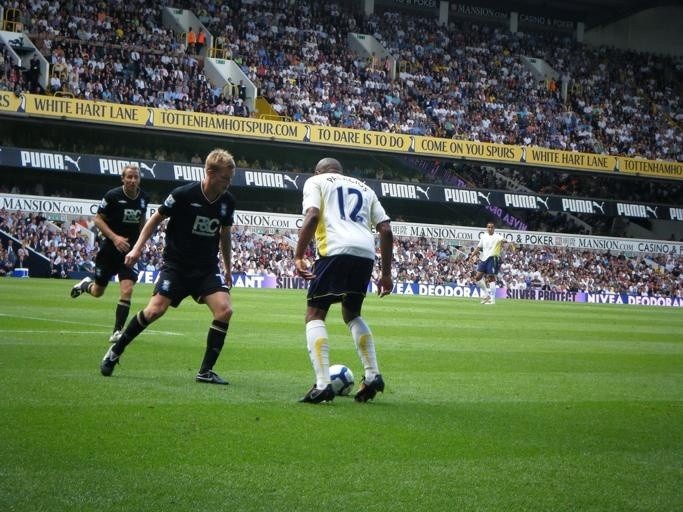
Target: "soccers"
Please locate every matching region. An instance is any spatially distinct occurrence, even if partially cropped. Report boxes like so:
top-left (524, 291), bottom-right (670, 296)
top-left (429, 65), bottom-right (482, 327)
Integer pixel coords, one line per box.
top-left (329, 363), bottom-right (354, 396)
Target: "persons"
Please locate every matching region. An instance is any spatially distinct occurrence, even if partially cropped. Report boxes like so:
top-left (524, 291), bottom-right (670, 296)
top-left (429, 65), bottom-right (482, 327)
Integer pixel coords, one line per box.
top-left (292, 158), bottom-right (395, 404)
top-left (1, 206), bottom-right (171, 278)
top-left (215, 224), bottom-right (316, 277)
top-left (396, 164), bottom-right (682, 241)
top-left (240, 1), bottom-right (683, 163)
top-left (236, 151), bottom-right (394, 180)
top-left (70, 165), bottom-right (146, 343)
top-left (1, 1), bottom-right (250, 117)
top-left (499, 241), bottom-right (683, 297)
top-left (100, 148), bottom-right (232, 386)
top-left (370, 236), bottom-right (490, 288)
top-left (468, 222), bottom-right (507, 305)
top-left (2, 128), bottom-right (207, 164)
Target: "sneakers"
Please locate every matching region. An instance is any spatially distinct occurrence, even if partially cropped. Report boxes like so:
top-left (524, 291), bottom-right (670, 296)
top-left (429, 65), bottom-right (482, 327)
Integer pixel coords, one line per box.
top-left (195, 371), bottom-right (229, 385)
top-left (109, 331), bottom-right (122, 342)
top-left (353, 374), bottom-right (385, 403)
top-left (297, 384), bottom-right (335, 402)
top-left (70, 276), bottom-right (91, 299)
top-left (100, 343), bottom-right (121, 376)
top-left (481, 296), bottom-right (495, 305)
